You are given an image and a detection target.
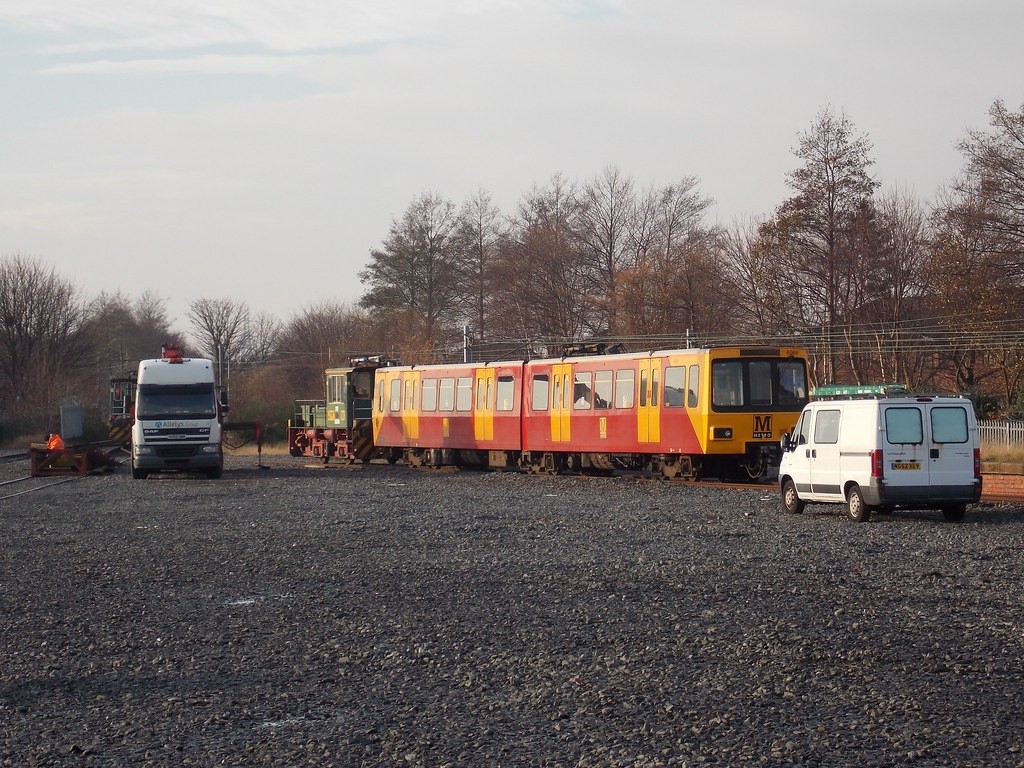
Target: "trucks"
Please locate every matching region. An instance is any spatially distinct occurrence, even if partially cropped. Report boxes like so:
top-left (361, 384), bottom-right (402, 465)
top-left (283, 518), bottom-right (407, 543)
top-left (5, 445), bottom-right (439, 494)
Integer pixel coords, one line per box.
top-left (131, 342), bottom-right (224, 478)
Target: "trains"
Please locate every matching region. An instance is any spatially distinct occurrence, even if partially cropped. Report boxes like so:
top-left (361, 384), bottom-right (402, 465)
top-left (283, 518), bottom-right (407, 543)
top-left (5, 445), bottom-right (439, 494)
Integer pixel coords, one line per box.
top-left (287, 345), bottom-right (812, 483)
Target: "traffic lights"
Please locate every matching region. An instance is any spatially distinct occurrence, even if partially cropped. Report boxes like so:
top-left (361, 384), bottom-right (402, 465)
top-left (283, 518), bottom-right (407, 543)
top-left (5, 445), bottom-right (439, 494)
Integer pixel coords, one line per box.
top-left (114, 384), bottom-right (122, 402)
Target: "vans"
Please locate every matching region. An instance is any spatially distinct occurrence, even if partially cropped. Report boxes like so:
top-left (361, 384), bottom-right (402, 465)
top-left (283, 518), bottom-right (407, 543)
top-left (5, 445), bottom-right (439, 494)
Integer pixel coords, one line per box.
top-left (778, 385), bottom-right (983, 523)
